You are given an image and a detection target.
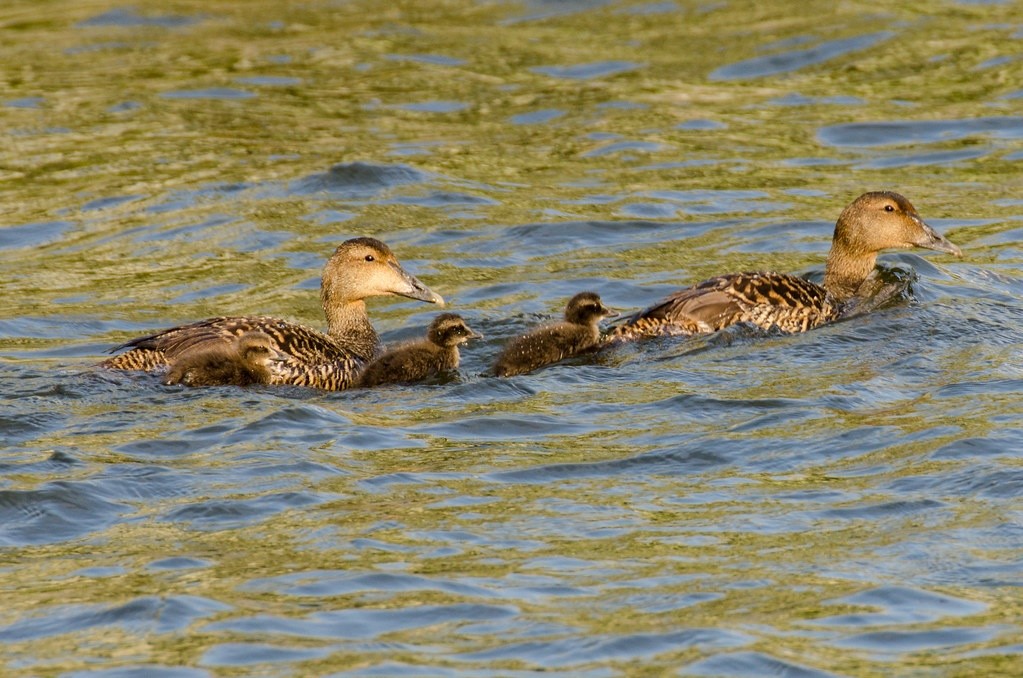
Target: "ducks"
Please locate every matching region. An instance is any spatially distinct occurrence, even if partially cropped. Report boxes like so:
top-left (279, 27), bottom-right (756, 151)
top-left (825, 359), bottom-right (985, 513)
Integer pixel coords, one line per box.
top-left (85, 235), bottom-right (486, 390)
top-left (476, 189), bottom-right (966, 381)
